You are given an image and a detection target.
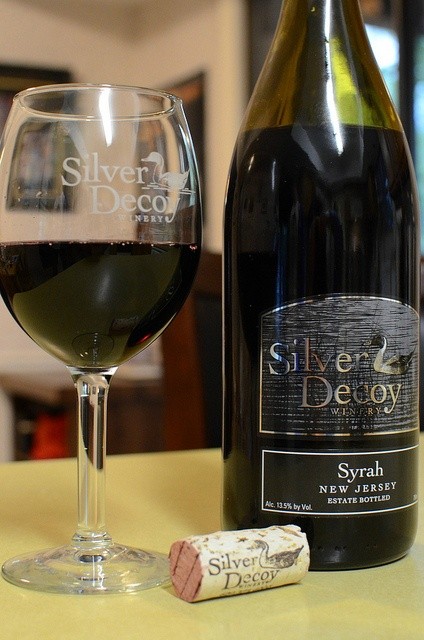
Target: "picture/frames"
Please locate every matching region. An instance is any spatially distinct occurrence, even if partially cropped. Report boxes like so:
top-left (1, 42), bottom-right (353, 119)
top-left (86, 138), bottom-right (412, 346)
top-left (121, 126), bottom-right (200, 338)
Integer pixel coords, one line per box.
top-left (2, 62), bottom-right (79, 214)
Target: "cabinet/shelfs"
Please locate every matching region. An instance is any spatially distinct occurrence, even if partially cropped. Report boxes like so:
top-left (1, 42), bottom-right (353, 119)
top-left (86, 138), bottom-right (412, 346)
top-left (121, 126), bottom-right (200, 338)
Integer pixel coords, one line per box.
top-left (0, 368), bottom-right (163, 460)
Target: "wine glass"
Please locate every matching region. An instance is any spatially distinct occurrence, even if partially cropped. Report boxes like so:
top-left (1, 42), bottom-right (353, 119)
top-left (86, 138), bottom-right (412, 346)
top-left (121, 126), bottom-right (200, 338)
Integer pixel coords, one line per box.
top-left (0, 81), bottom-right (204, 596)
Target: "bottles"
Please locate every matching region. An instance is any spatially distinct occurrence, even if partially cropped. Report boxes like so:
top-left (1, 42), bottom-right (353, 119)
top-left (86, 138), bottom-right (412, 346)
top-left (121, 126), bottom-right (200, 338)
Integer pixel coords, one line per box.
top-left (218, 0), bottom-right (420, 573)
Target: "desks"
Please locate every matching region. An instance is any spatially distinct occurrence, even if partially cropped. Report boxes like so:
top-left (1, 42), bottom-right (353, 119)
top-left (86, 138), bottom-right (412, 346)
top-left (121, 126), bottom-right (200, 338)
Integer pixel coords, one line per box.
top-left (1, 427), bottom-right (424, 640)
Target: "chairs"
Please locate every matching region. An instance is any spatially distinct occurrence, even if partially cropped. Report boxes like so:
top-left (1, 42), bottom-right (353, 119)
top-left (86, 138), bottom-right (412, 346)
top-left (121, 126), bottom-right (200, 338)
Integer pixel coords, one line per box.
top-left (160, 250), bottom-right (222, 450)
